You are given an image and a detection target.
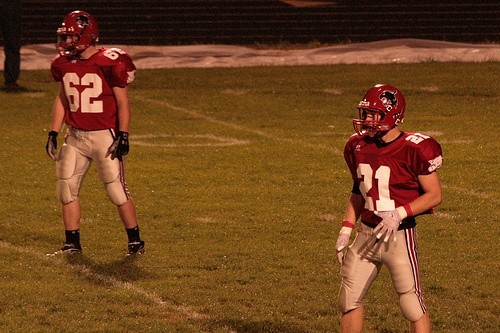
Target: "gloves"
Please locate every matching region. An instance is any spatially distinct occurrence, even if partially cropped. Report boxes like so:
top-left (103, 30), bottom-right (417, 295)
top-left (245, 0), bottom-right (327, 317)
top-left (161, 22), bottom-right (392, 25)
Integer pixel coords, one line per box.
top-left (46, 130), bottom-right (59, 161)
top-left (372, 203), bottom-right (413, 243)
top-left (108, 131), bottom-right (130, 162)
top-left (336, 221), bottom-right (355, 264)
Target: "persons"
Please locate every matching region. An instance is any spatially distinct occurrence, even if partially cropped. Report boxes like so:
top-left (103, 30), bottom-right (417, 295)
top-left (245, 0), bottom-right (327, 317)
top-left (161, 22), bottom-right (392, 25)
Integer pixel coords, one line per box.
top-left (0, 0), bottom-right (28, 92)
top-left (46, 10), bottom-right (146, 261)
top-left (335, 83), bottom-right (444, 333)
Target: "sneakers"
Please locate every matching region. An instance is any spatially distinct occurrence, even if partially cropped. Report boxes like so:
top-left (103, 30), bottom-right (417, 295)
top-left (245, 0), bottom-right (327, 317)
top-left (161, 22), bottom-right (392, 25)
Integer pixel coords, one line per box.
top-left (46, 241), bottom-right (83, 259)
top-left (126, 241), bottom-right (146, 260)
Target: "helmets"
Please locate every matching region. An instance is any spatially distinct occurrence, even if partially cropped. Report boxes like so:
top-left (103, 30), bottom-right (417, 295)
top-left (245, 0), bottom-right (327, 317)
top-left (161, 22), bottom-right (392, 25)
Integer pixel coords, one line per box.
top-left (357, 83), bottom-right (407, 132)
top-left (55, 10), bottom-right (100, 51)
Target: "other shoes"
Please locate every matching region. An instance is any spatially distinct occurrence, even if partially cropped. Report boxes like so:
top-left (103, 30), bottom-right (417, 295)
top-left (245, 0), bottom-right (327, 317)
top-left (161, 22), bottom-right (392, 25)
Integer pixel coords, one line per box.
top-left (3, 81), bottom-right (25, 92)
